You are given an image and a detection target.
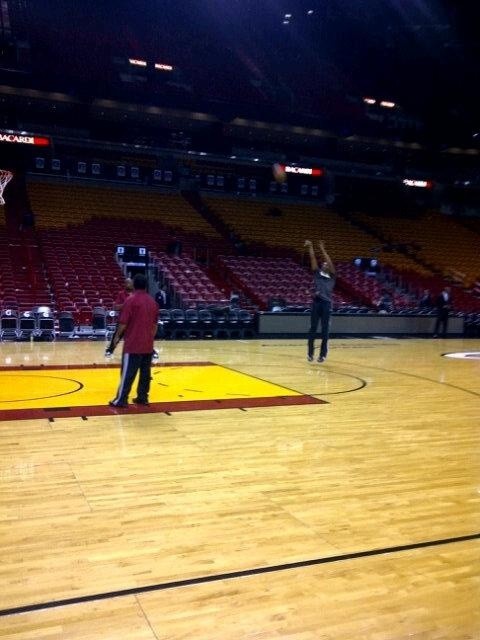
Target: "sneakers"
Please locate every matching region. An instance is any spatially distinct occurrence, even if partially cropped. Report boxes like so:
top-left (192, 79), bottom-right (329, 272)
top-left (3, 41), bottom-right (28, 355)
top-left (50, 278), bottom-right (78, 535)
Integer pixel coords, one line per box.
top-left (318, 356), bottom-right (325, 362)
top-left (306, 355), bottom-right (314, 360)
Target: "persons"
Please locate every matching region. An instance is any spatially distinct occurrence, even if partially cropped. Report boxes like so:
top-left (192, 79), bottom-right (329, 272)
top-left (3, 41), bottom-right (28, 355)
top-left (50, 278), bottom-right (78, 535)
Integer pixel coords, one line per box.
top-left (155, 284), bottom-right (171, 310)
top-left (432, 287), bottom-right (452, 339)
top-left (417, 288), bottom-right (432, 305)
top-left (304, 239), bottom-right (337, 364)
top-left (105, 277), bottom-right (158, 360)
top-left (109, 274), bottom-right (160, 408)
